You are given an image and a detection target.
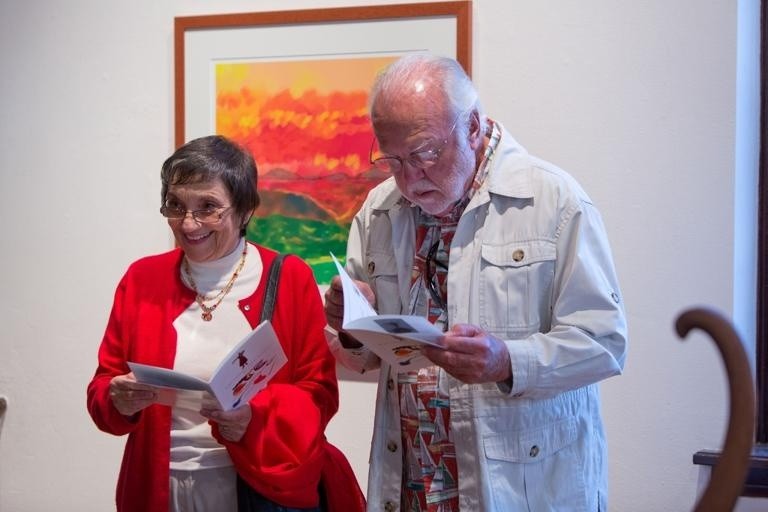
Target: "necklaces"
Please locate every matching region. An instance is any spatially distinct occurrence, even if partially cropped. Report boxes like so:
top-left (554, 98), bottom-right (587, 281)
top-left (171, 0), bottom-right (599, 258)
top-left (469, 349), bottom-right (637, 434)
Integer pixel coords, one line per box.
top-left (182, 239), bottom-right (248, 320)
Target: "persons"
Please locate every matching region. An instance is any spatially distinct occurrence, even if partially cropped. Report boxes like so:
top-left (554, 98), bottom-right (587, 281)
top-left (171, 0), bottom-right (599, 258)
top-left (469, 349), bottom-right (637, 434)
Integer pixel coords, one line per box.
top-left (86, 135), bottom-right (367, 512)
top-left (323, 51), bottom-right (629, 512)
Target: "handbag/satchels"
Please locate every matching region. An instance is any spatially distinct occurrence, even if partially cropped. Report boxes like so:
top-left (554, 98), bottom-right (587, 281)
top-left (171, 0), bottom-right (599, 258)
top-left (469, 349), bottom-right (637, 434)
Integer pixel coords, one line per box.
top-left (237, 469), bottom-right (329, 512)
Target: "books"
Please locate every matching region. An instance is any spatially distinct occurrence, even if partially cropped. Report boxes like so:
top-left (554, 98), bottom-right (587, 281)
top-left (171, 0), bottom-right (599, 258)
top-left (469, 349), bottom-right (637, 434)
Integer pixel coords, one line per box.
top-left (121, 320), bottom-right (289, 415)
top-left (328, 248), bottom-right (449, 376)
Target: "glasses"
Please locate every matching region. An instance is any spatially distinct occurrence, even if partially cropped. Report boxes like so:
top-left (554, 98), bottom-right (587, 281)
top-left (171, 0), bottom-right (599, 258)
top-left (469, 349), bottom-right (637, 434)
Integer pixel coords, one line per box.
top-left (160, 200), bottom-right (234, 224)
top-left (426, 241), bottom-right (446, 310)
top-left (370, 112), bottom-right (462, 173)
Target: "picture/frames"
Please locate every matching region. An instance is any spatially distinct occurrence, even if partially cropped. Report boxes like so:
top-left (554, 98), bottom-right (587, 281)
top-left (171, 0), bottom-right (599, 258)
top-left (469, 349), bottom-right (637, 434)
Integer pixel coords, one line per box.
top-left (173, 1), bottom-right (472, 312)
